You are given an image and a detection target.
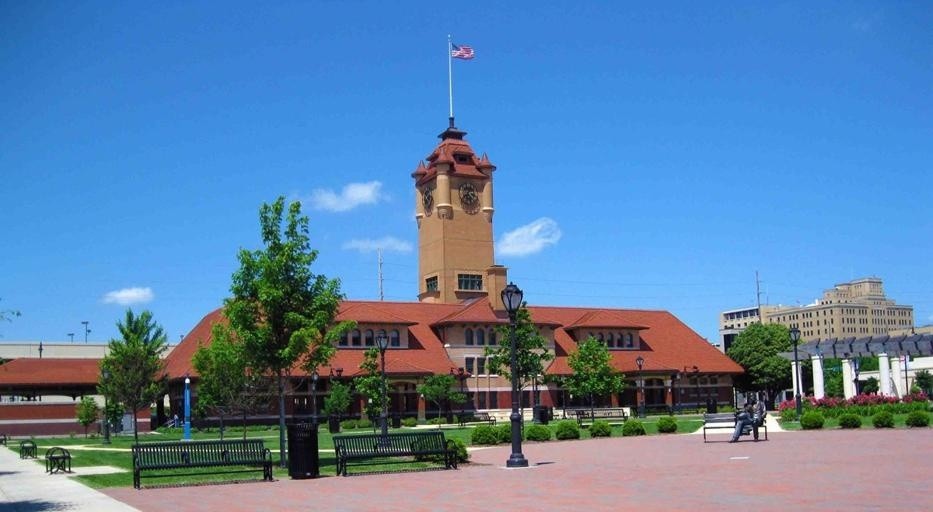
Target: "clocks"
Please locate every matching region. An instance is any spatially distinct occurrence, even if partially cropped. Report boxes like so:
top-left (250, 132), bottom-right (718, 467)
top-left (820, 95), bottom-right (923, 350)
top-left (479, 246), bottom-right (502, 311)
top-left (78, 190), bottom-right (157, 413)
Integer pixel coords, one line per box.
top-left (458, 181), bottom-right (478, 206)
top-left (420, 186), bottom-right (433, 209)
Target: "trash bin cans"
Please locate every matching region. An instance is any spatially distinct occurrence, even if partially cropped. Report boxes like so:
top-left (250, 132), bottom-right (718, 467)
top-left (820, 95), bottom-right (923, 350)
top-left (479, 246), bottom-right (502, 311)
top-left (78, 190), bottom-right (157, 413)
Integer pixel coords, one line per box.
top-left (286, 421), bottom-right (320, 479)
top-left (329, 414), bottom-right (340, 433)
top-left (392, 412), bottom-right (400, 428)
top-left (707, 397), bottom-right (717, 414)
top-left (534, 405), bottom-right (549, 425)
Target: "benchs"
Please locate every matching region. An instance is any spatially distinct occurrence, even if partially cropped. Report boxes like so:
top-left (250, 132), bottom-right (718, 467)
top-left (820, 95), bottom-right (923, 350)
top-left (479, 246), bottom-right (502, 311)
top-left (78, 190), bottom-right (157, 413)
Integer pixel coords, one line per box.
top-left (18, 440), bottom-right (37, 463)
top-left (458, 410), bottom-right (494, 427)
top-left (331, 432), bottom-right (458, 476)
top-left (45, 446), bottom-right (73, 473)
top-left (702, 412), bottom-right (768, 444)
top-left (574, 408), bottom-right (625, 429)
top-left (131, 439), bottom-right (274, 491)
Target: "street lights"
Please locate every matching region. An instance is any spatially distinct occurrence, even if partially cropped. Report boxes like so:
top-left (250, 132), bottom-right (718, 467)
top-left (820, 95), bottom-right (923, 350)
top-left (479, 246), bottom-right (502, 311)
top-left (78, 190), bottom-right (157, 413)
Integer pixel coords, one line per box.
top-left (676, 372), bottom-right (682, 414)
top-left (82, 320), bottom-right (91, 344)
top-left (458, 367), bottom-right (465, 394)
top-left (67, 333), bottom-right (74, 345)
top-left (375, 328), bottom-right (393, 447)
top-left (499, 279), bottom-right (530, 467)
top-left (310, 368), bottom-right (320, 431)
top-left (183, 374), bottom-right (192, 440)
top-left (180, 334), bottom-right (184, 343)
top-left (635, 356), bottom-right (647, 418)
top-left (851, 357), bottom-right (861, 397)
top-left (327, 369), bottom-right (334, 383)
top-left (789, 323), bottom-right (806, 418)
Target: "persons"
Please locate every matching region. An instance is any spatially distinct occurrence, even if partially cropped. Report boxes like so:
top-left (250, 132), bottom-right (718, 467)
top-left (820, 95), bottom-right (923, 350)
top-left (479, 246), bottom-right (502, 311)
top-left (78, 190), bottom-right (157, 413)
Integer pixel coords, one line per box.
top-left (727, 403), bottom-right (752, 443)
top-left (745, 391), bottom-right (768, 442)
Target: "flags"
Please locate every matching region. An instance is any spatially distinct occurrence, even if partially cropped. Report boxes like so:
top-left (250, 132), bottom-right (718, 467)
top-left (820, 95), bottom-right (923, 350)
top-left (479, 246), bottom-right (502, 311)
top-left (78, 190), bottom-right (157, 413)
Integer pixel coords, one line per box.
top-left (446, 33), bottom-right (476, 61)
